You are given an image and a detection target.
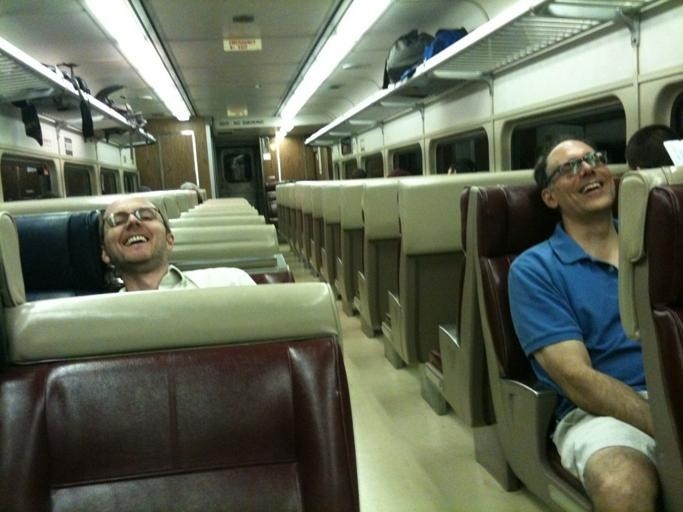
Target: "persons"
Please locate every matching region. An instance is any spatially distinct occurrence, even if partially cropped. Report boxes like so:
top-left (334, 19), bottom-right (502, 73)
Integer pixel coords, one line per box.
top-left (506, 138), bottom-right (662, 512)
top-left (623, 123), bottom-right (678, 170)
top-left (99, 196), bottom-right (259, 292)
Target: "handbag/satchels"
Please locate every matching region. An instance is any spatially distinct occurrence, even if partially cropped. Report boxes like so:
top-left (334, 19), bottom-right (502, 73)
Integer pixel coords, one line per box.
top-left (38, 62), bottom-right (91, 94)
top-left (382, 29), bottom-right (434, 89)
top-left (401, 27), bottom-right (467, 79)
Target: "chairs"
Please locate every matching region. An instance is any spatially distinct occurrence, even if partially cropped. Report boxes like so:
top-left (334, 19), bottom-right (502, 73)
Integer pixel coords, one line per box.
top-left (1, 159), bottom-right (682, 511)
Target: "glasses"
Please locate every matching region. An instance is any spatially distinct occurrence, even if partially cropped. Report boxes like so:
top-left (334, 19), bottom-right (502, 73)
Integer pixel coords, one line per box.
top-left (542, 149), bottom-right (608, 186)
top-left (103, 206), bottom-right (168, 231)
